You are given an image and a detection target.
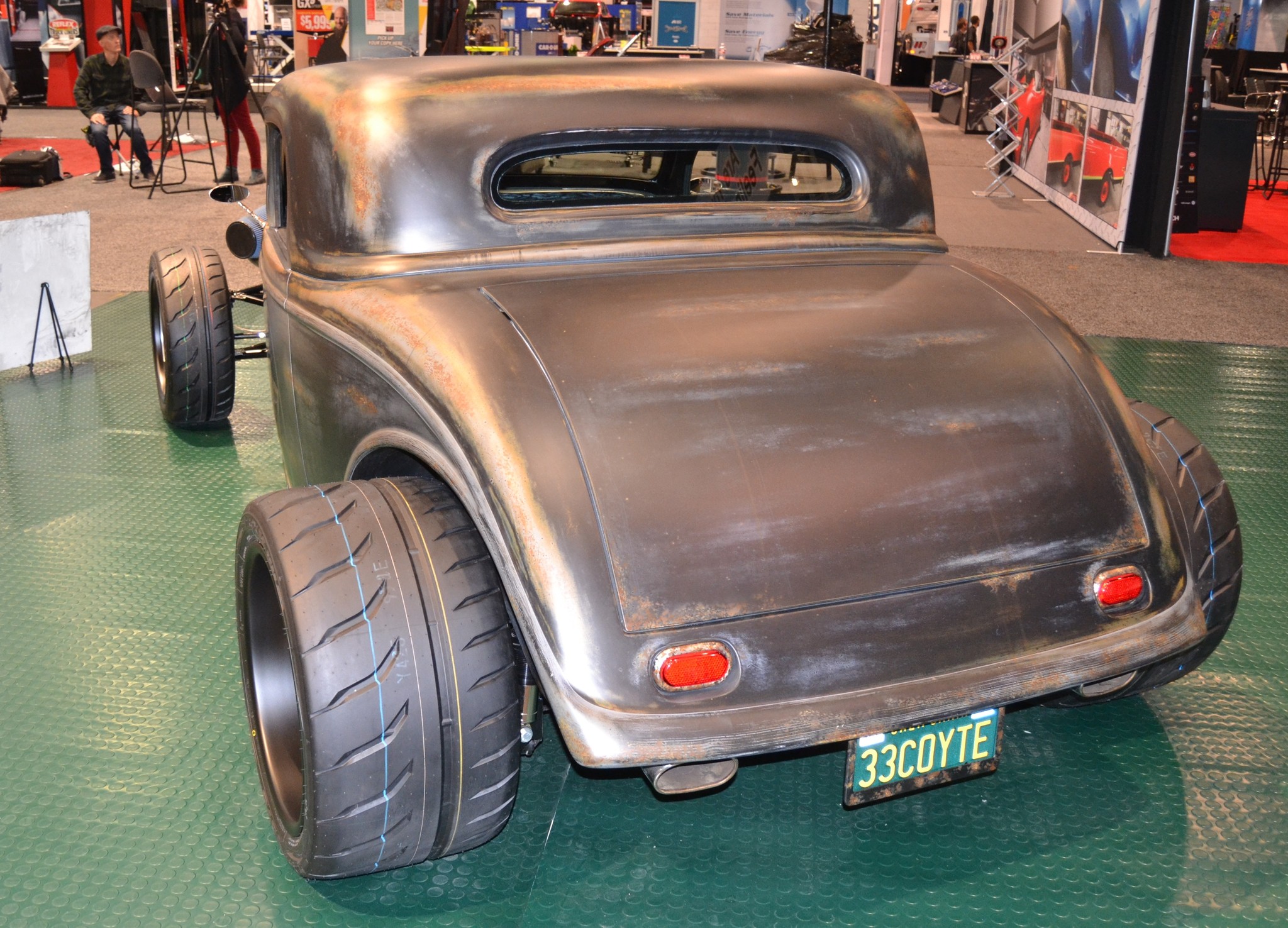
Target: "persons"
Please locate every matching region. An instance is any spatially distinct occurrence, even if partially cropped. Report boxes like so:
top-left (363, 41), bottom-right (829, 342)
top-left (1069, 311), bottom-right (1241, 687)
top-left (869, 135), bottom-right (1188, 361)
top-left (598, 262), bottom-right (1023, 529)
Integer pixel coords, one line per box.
top-left (315, 6), bottom-right (348, 66)
top-left (73, 24), bottom-right (161, 184)
top-left (204, 0), bottom-right (265, 186)
top-left (965, 16), bottom-right (980, 56)
top-left (949, 18), bottom-right (970, 56)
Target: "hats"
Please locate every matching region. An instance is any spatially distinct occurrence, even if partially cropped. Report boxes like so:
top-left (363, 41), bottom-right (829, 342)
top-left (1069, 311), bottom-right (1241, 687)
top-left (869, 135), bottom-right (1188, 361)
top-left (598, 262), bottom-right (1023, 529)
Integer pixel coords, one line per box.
top-left (96, 25), bottom-right (122, 40)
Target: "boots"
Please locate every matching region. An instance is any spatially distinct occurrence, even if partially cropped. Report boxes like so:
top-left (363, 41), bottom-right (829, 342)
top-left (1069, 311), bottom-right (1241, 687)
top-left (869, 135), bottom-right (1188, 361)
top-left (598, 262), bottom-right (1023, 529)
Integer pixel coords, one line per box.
top-left (213, 165), bottom-right (238, 181)
top-left (243, 168), bottom-right (266, 185)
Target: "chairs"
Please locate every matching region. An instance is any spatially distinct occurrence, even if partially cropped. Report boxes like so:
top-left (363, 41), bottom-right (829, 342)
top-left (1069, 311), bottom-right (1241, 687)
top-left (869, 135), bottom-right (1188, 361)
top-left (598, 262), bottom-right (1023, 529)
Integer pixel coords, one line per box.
top-left (129, 50), bottom-right (220, 194)
top-left (1210, 70), bottom-right (1288, 201)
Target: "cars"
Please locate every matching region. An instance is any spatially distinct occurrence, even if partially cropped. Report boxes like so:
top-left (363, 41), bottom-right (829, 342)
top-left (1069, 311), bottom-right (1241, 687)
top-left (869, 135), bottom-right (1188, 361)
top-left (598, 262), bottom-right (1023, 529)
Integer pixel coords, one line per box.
top-left (1009, 68), bottom-right (1125, 205)
top-left (146, 56), bottom-right (1239, 881)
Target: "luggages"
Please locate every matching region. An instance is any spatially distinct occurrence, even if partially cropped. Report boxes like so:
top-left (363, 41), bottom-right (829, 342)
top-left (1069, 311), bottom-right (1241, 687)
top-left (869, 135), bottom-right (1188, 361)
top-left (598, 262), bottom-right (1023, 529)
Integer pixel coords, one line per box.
top-left (1, 149), bottom-right (71, 188)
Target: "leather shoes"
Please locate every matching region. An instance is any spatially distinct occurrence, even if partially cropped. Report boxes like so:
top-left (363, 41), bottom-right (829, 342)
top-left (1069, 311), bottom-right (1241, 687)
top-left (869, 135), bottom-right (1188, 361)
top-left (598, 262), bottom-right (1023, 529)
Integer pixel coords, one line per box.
top-left (91, 171), bottom-right (115, 183)
top-left (142, 170), bottom-right (161, 182)
